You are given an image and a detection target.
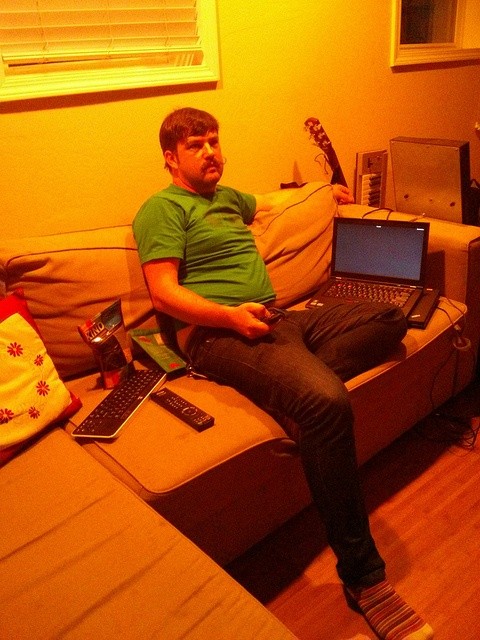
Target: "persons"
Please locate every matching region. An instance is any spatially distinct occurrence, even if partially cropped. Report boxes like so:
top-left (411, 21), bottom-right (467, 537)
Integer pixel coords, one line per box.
top-left (132, 107), bottom-right (435, 640)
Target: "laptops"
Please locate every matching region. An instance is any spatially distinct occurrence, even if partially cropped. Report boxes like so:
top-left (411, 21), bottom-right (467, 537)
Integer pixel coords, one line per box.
top-left (407, 287), bottom-right (441, 329)
top-left (305, 216), bottom-right (430, 319)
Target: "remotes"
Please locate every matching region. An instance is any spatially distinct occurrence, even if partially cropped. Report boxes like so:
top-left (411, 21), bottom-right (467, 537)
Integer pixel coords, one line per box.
top-left (149, 387), bottom-right (215, 433)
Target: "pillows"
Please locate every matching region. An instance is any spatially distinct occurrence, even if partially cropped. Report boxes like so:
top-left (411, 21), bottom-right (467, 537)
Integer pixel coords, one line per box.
top-left (0, 287), bottom-right (81, 468)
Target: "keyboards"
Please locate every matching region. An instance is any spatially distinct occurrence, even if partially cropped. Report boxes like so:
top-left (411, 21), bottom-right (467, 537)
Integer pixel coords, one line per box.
top-left (71, 368), bottom-right (168, 439)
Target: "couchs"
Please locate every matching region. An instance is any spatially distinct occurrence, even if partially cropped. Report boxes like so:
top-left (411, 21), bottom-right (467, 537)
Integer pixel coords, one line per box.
top-left (2, 183), bottom-right (480, 570)
top-left (1, 282), bottom-right (300, 640)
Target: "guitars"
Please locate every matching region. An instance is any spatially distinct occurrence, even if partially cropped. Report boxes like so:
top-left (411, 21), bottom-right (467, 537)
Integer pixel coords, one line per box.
top-left (304, 118), bottom-right (347, 186)
